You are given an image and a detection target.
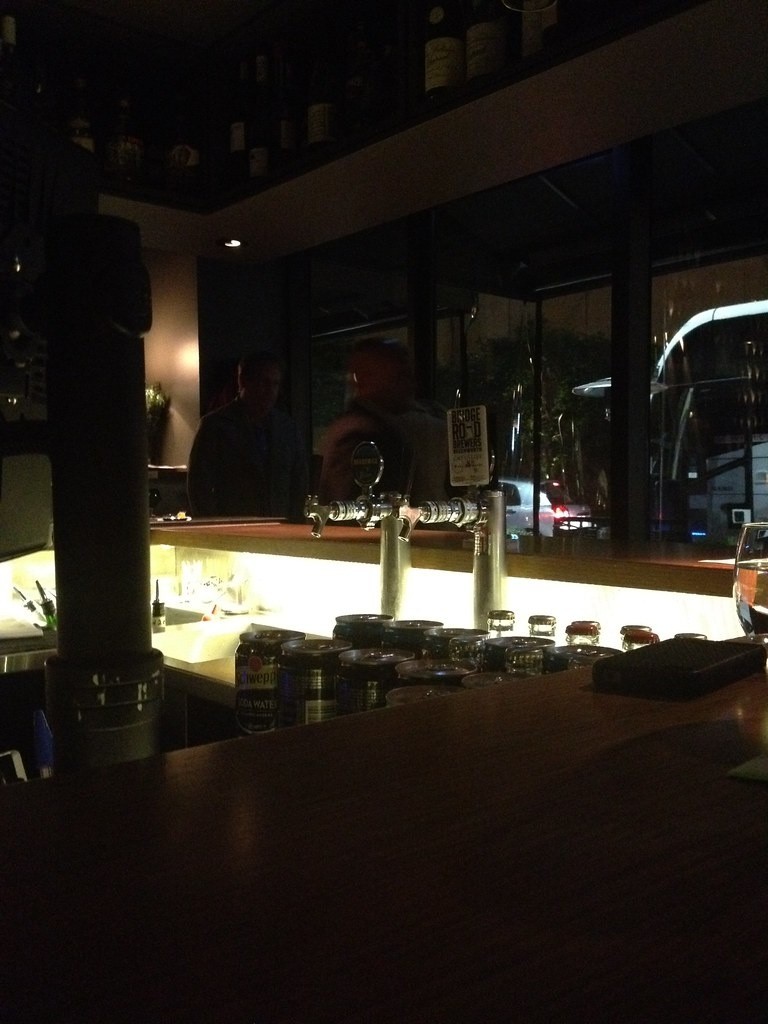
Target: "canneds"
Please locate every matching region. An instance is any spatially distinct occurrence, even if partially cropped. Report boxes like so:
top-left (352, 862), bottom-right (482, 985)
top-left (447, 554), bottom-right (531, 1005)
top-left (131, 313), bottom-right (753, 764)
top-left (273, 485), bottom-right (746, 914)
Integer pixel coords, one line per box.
top-left (232, 606), bottom-right (714, 739)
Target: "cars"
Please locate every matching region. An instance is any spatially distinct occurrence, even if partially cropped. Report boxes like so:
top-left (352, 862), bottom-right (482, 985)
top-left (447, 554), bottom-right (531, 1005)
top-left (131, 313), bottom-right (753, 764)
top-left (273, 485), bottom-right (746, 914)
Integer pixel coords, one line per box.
top-left (477, 475), bottom-right (595, 538)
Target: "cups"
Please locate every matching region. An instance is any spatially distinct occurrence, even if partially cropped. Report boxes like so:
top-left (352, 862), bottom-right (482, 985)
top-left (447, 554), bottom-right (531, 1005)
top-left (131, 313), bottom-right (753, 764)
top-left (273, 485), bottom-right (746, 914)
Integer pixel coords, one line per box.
top-left (731, 522), bottom-right (768, 646)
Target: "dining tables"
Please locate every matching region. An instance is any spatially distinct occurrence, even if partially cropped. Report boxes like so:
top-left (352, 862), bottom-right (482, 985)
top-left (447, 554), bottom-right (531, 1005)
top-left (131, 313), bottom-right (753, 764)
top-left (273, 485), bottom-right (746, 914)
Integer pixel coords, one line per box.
top-left (0, 621), bottom-right (768, 1023)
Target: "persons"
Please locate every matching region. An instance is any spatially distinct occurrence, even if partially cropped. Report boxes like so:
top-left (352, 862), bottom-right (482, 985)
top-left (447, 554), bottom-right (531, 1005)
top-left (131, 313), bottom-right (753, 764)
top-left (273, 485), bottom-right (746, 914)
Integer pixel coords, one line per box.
top-left (186, 349), bottom-right (312, 529)
top-left (316, 337), bottom-right (457, 533)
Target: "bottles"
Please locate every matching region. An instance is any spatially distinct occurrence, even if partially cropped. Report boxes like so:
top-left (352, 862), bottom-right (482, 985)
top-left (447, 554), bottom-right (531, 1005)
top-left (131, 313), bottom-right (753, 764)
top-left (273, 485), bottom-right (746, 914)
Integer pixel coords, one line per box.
top-left (4, 2), bottom-right (592, 190)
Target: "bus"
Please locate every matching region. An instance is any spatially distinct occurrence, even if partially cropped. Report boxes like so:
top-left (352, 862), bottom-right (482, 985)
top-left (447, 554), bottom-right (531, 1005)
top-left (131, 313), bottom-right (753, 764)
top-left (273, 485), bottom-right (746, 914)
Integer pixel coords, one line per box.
top-left (639, 299), bottom-right (768, 557)
